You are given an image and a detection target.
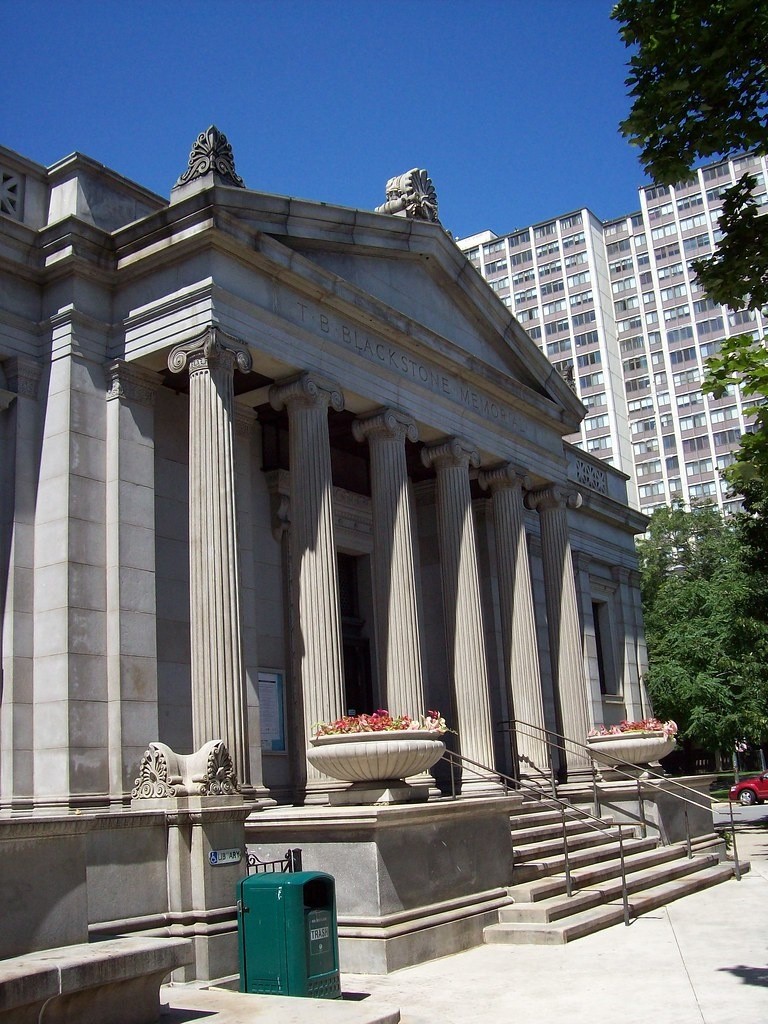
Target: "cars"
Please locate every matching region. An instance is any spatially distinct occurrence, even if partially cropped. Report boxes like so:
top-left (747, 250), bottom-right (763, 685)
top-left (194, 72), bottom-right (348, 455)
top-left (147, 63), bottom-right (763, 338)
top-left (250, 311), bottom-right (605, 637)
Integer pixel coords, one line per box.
top-left (729, 769), bottom-right (768, 805)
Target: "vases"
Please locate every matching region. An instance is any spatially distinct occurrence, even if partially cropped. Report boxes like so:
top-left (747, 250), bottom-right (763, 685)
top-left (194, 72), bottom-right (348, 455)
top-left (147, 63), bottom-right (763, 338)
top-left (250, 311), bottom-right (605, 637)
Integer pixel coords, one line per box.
top-left (307, 730), bottom-right (446, 807)
top-left (584, 731), bottom-right (676, 782)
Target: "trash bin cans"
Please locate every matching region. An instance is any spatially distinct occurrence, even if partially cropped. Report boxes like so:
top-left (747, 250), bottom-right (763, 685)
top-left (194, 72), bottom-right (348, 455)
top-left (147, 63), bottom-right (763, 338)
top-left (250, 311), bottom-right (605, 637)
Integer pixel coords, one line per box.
top-left (234, 871), bottom-right (342, 1000)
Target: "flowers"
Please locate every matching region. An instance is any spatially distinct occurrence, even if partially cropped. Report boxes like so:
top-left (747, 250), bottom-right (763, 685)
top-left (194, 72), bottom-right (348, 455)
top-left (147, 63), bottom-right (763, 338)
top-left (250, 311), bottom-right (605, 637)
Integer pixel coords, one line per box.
top-left (310, 709), bottom-right (458, 737)
top-left (587, 715), bottom-right (678, 743)
